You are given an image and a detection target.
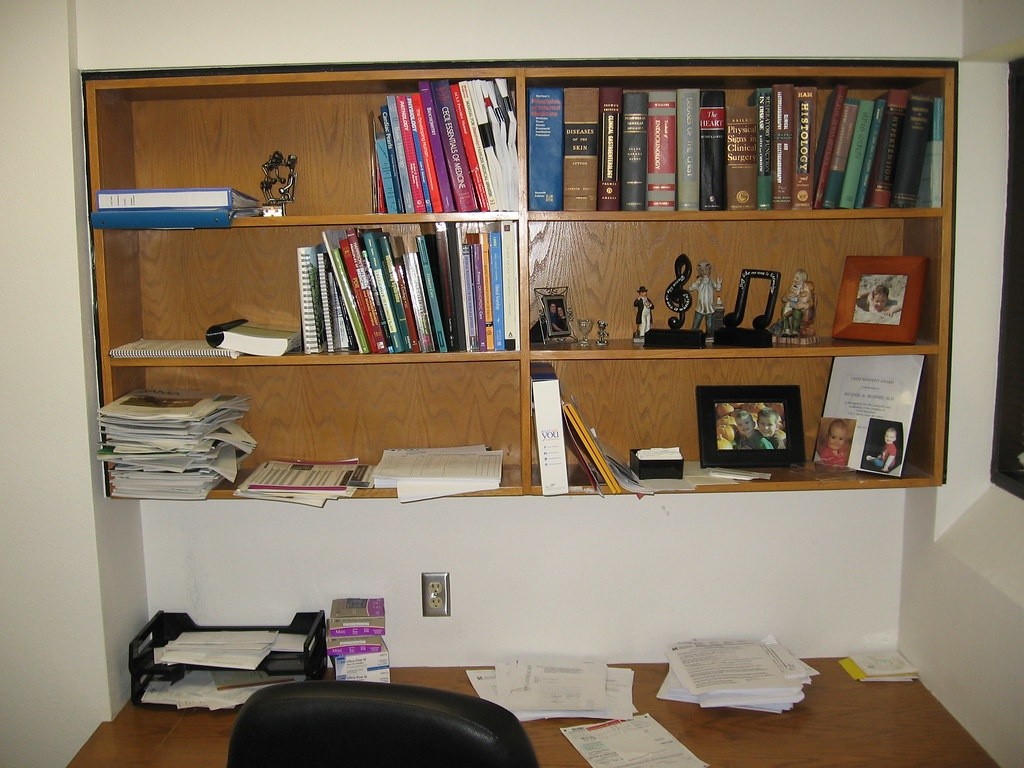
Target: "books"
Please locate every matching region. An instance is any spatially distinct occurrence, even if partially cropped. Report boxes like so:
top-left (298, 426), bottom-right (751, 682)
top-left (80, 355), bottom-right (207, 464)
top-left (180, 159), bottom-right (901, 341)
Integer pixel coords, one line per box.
top-left (108, 316), bottom-right (298, 360)
top-left (293, 218), bottom-right (522, 357)
top-left (528, 72), bottom-right (946, 212)
top-left (369, 77), bottom-right (520, 215)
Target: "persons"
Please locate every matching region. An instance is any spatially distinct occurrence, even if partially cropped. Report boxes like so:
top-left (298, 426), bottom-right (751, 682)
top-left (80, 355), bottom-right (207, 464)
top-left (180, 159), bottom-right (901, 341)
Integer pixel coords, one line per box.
top-left (550, 303), bottom-right (563, 331)
top-left (867, 427), bottom-right (898, 472)
top-left (733, 410), bottom-right (774, 449)
top-left (632, 286), bottom-right (655, 339)
top-left (861, 285), bottom-right (903, 317)
top-left (756, 407), bottom-right (786, 449)
top-left (689, 261), bottom-right (723, 343)
top-left (778, 271), bottom-right (813, 337)
top-left (819, 420), bottom-right (849, 466)
top-left (556, 306), bottom-right (566, 331)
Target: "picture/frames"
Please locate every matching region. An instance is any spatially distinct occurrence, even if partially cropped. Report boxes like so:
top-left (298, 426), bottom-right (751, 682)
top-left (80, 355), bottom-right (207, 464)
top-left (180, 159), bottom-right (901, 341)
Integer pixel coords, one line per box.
top-left (832, 255), bottom-right (929, 345)
top-left (696, 385), bottom-right (807, 470)
top-left (542, 295), bottom-right (572, 337)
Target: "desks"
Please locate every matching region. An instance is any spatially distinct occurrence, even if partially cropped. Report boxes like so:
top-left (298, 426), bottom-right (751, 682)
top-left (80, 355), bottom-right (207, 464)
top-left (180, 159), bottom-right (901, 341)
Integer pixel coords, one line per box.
top-left (67, 657), bottom-right (1000, 768)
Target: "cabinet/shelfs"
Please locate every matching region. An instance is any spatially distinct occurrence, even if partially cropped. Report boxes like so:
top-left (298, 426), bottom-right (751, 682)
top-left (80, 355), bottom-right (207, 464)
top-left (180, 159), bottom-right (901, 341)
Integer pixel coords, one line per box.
top-left (82, 58), bottom-right (960, 499)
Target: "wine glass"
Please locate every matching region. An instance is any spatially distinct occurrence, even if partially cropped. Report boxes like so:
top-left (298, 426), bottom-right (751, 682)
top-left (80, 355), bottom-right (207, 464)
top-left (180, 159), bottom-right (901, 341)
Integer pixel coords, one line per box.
top-left (577, 320), bottom-right (594, 346)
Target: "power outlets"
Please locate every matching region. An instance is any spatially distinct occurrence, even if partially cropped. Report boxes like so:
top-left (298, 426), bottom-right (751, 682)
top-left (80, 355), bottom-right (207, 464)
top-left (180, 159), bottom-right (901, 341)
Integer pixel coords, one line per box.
top-left (422, 571), bottom-right (452, 617)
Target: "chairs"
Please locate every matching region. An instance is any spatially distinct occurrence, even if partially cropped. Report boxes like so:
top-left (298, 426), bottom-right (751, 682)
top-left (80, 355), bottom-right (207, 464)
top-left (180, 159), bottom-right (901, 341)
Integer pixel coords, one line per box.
top-left (225, 682), bottom-right (540, 768)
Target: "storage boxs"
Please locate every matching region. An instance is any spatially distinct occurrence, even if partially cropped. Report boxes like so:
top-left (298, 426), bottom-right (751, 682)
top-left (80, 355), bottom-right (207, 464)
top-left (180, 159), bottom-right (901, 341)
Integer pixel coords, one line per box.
top-left (326, 628), bottom-right (382, 656)
top-left (530, 362), bottom-right (569, 498)
top-left (329, 617), bottom-right (386, 637)
top-left (628, 448), bottom-right (686, 480)
top-left (329, 637), bottom-right (389, 683)
top-left (330, 598), bottom-right (385, 618)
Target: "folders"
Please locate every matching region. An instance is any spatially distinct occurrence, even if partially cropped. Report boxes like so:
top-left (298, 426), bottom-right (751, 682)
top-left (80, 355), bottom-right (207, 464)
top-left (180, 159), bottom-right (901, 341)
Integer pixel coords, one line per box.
top-left (88, 183), bottom-right (265, 235)
